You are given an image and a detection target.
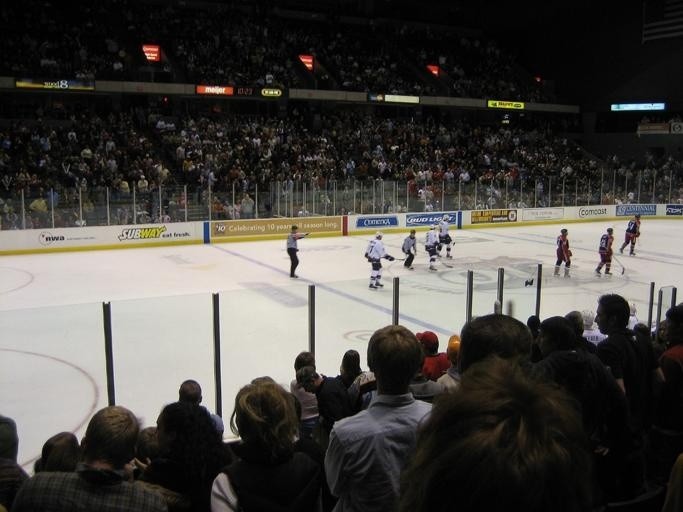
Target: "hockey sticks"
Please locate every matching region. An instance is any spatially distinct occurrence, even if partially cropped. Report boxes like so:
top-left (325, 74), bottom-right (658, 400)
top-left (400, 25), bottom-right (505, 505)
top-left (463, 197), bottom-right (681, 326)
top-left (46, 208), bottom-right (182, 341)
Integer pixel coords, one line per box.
top-left (381, 259), bottom-right (405, 261)
top-left (613, 256), bottom-right (624, 275)
top-left (438, 257), bottom-right (453, 268)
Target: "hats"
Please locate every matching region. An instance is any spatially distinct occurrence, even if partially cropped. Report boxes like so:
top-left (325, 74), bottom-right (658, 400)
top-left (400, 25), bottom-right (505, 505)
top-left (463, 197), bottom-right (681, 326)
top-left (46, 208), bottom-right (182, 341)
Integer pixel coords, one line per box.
top-left (448, 335), bottom-right (460, 352)
top-left (416, 331), bottom-right (438, 348)
top-left (295, 366), bottom-right (314, 388)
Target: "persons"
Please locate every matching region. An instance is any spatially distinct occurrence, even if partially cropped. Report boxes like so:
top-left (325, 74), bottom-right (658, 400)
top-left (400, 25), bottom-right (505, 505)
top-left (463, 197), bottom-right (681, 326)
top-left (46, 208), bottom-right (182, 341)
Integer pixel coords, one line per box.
top-left (287, 224), bottom-right (310, 278)
top-left (425, 224), bottom-right (439, 270)
top-left (595, 227), bottom-right (614, 276)
top-left (365, 231), bottom-right (394, 288)
top-left (401, 230), bottom-right (418, 267)
top-left (0, 380), bottom-right (224, 511)
top-left (553, 229), bottom-right (572, 277)
top-left (619, 213), bottom-right (641, 256)
top-left (436, 215), bottom-right (455, 257)
top-left (0, 0), bottom-right (683, 226)
top-left (210, 294), bottom-right (683, 512)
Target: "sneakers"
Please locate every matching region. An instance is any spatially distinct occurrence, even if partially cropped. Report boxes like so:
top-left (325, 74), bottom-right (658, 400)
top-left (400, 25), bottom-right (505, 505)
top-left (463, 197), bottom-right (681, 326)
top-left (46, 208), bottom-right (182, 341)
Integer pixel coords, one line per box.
top-left (369, 283), bottom-right (382, 288)
